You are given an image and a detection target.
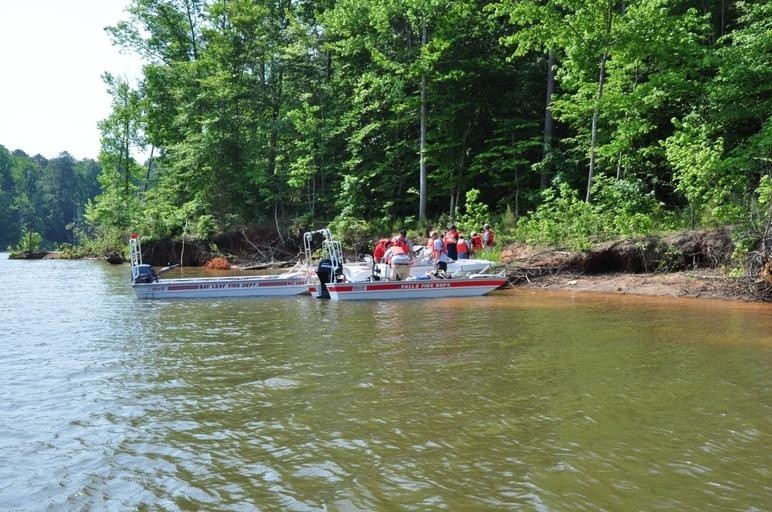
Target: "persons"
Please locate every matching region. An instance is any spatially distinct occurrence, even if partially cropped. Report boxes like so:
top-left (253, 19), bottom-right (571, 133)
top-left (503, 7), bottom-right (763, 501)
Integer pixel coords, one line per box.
top-left (427, 223), bottom-right (494, 260)
top-left (373, 230), bottom-right (414, 281)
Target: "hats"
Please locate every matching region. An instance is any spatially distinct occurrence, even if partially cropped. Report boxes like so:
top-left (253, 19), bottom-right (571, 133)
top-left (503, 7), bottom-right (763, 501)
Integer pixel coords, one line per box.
top-left (447, 224), bottom-right (456, 230)
top-left (400, 229), bottom-right (408, 238)
